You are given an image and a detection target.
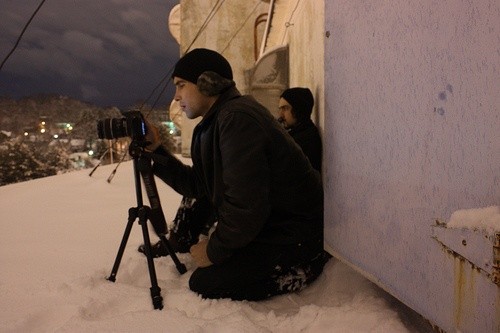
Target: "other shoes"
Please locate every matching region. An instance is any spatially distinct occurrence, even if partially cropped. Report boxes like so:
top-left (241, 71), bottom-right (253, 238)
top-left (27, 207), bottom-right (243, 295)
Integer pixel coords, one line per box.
top-left (138, 237), bottom-right (190, 257)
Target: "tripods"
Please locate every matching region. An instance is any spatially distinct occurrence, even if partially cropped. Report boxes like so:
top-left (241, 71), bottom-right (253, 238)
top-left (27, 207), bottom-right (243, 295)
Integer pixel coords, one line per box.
top-left (107, 138), bottom-right (187, 309)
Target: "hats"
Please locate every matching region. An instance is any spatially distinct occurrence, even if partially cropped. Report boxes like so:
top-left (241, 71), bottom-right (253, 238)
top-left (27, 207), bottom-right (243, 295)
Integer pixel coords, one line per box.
top-left (171, 48), bottom-right (233, 87)
top-left (280, 87), bottom-right (315, 121)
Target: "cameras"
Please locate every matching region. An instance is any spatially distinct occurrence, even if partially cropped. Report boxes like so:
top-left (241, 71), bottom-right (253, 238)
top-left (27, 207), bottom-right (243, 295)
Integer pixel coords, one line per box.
top-left (97, 111), bottom-right (146, 138)
